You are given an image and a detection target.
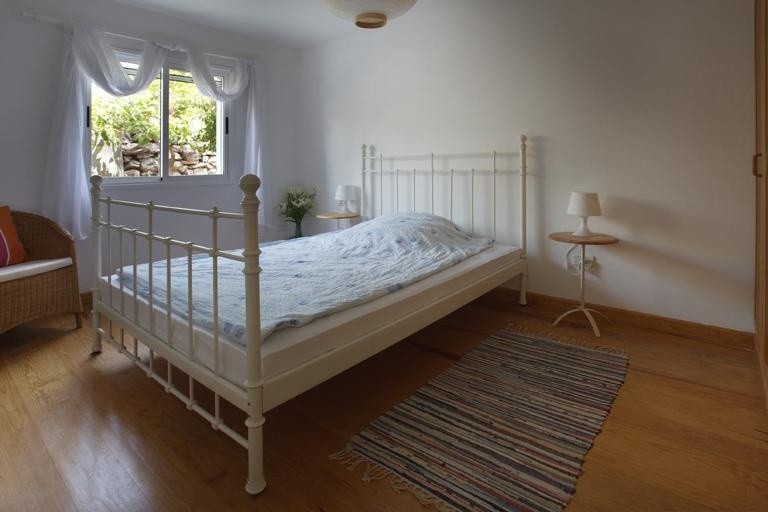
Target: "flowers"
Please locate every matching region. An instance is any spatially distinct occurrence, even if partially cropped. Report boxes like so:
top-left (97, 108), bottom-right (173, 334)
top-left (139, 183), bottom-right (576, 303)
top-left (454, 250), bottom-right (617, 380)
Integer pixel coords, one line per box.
top-left (275, 182), bottom-right (319, 224)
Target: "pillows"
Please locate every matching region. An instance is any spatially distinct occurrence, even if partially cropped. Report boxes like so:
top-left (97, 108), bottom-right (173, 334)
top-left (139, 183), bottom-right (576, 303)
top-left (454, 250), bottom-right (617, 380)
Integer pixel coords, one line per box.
top-left (0, 205), bottom-right (29, 268)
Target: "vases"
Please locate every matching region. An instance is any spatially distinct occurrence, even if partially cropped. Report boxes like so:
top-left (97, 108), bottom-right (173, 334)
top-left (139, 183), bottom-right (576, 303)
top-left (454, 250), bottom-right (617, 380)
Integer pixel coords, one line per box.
top-left (288, 224), bottom-right (306, 240)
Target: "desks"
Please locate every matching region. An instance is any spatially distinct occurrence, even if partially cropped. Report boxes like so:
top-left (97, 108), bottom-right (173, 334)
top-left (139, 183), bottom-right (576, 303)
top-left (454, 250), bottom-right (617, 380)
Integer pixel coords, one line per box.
top-left (316, 212), bottom-right (361, 231)
top-left (546, 232), bottom-right (621, 338)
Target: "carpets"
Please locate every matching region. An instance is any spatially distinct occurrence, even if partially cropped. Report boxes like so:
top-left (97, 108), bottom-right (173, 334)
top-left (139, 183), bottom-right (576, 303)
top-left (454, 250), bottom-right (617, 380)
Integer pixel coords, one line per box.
top-left (327, 321), bottom-right (631, 510)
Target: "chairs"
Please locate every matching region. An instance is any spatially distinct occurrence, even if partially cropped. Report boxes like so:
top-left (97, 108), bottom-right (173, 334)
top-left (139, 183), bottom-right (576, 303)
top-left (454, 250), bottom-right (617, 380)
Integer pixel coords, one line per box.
top-left (0, 211), bottom-right (86, 336)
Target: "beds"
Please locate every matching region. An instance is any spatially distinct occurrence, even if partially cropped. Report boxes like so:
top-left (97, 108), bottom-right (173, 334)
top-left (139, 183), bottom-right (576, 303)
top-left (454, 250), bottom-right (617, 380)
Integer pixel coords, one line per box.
top-left (88, 133), bottom-right (528, 495)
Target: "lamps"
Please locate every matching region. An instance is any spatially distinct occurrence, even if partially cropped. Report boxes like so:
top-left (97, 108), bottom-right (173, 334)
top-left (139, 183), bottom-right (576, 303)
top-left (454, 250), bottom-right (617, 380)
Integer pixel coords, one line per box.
top-left (326, 0), bottom-right (418, 28)
top-left (565, 191), bottom-right (602, 238)
top-left (334, 184), bottom-right (356, 215)
